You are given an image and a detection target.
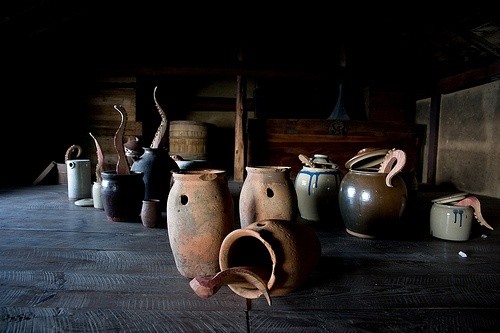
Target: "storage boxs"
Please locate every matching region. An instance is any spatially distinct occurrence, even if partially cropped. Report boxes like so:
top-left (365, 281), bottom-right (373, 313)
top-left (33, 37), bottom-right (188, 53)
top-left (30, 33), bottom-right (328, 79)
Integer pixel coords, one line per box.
top-left (64, 76), bottom-right (137, 182)
top-left (246, 118), bottom-right (427, 189)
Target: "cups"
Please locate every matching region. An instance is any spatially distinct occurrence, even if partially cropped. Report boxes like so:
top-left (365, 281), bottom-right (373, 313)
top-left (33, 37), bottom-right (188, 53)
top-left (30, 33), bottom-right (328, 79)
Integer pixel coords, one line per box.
top-left (430, 203), bottom-right (473, 241)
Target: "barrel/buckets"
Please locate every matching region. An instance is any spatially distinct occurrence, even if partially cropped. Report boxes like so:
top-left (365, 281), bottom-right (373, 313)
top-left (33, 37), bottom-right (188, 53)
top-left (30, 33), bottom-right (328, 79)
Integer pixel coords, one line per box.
top-left (169, 120), bottom-right (208, 160)
